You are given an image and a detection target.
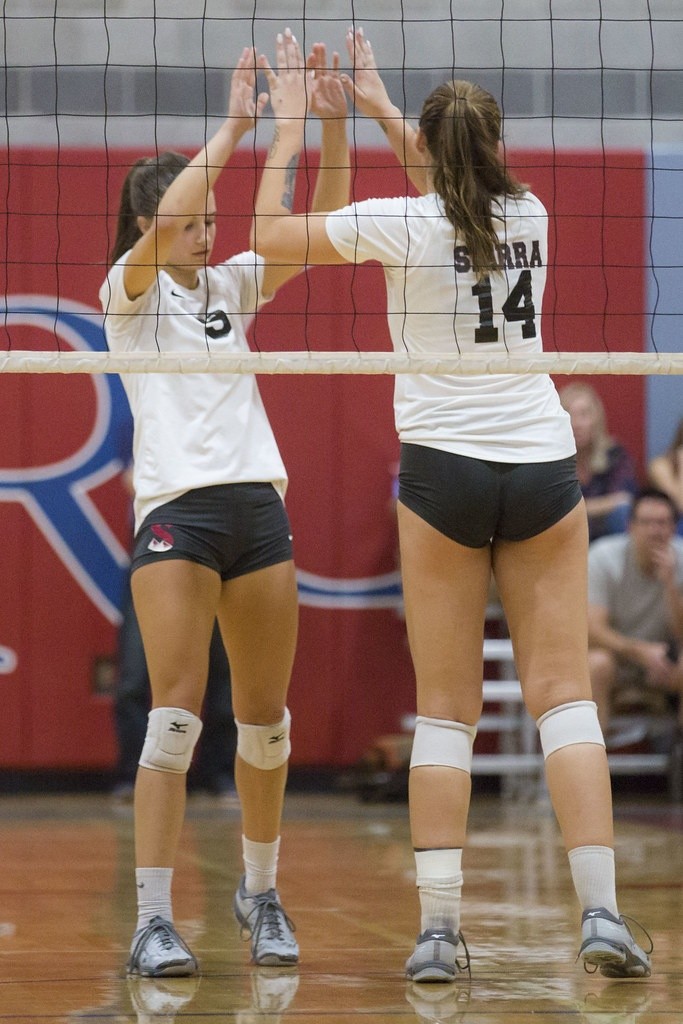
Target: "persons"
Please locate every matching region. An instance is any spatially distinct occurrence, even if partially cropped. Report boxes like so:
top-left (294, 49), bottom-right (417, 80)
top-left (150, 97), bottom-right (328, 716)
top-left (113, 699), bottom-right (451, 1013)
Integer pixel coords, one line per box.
top-left (115, 424), bottom-right (238, 804)
top-left (250, 26), bottom-right (653, 980)
top-left (554, 382), bottom-right (683, 735)
top-left (100, 44), bottom-right (350, 975)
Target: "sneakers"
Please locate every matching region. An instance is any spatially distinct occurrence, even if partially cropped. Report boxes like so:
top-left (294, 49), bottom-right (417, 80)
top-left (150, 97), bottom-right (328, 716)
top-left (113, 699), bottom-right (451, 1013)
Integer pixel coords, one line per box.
top-left (129, 982), bottom-right (195, 1018)
top-left (572, 906), bottom-right (653, 978)
top-left (253, 974), bottom-right (300, 1013)
top-left (404, 927), bottom-right (471, 981)
top-left (125, 916), bottom-right (197, 977)
top-left (232, 873), bottom-right (300, 965)
top-left (583, 988), bottom-right (648, 1022)
top-left (406, 986), bottom-right (459, 1019)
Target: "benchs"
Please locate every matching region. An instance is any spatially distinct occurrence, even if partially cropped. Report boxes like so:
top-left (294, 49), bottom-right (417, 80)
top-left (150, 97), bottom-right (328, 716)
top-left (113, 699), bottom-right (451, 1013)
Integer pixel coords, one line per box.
top-left (400, 594), bottom-right (683, 802)
